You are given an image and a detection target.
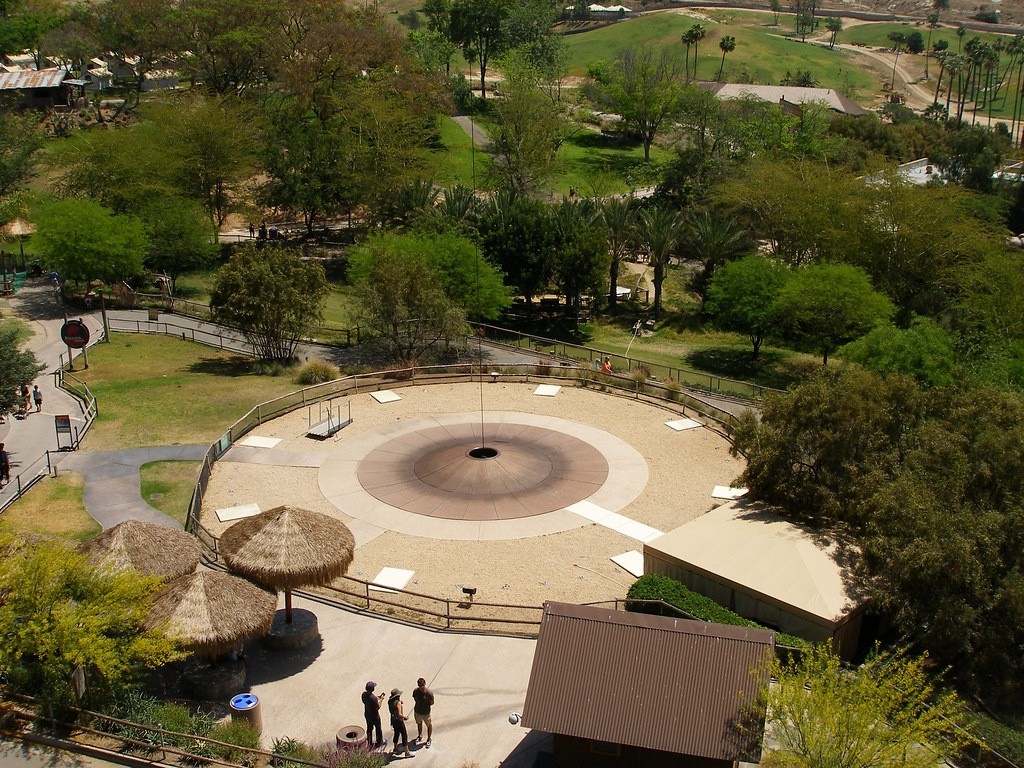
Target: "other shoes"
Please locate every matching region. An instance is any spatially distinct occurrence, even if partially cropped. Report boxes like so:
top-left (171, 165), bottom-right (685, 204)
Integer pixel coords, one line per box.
top-left (377, 738), bottom-right (388, 746)
top-left (426, 738), bottom-right (431, 748)
top-left (393, 745), bottom-right (399, 753)
top-left (414, 735), bottom-right (423, 742)
top-left (405, 752), bottom-right (415, 757)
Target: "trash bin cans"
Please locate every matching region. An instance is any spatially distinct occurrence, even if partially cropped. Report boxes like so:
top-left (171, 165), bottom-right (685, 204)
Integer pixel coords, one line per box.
top-left (336, 725), bottom-right (366, 750)
top-left (230, 692), bottom-right (262, 736)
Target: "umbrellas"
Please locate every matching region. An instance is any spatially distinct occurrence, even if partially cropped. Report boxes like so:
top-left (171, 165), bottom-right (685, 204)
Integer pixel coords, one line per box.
top-left (0, 217), bottom-right (38, 267)
top-left (76, 519), bottom-right (201, 584)
top-left (217, 505), bottom-right (356, 625)
top-left (142, 571), bottom-right (277, 668)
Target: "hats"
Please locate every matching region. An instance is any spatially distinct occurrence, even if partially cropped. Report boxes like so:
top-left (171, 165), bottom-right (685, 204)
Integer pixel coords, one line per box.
top-left (366, 681), bottom-right (377, 690)
top-left (390, 689), bottom-right (403, 700)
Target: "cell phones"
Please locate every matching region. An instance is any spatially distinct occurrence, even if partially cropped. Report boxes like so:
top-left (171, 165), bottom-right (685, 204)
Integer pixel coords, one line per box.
top-left (379, 693), bottom-right (385, 698)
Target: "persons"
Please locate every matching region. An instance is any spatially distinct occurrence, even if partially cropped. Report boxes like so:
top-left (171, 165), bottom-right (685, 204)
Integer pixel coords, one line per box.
top-left (569, 185), bottom-right (580, 200)
top-left (21, 384), bottom-right (32, 409)
top-left (412, 678), bottom-right (434, 748)
top-left (249, 222), bottom-right (254, 237)
top-left (594, 357), bottom-right (614, 373)
top-left (33, 385), bottom-right (44, 411)
top-left (51, 269), bottom-right (58, 291)
top-left (0, 443), bottom-right (11, 489)
top-left (388, 689), bottom-right (415, 758)
top-left (362, 681), bottom-right (388, 747)
top-left (259, 221), bottom-right (266, 240)
top-left (479, 326), bottom-right (486, 342)
top-left (12, 387), bottom-right (28, 419)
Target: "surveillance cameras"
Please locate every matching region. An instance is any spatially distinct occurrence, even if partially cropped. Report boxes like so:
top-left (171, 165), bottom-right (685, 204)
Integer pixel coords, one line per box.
top-left (509, 715), bottom-right (519, 724)
